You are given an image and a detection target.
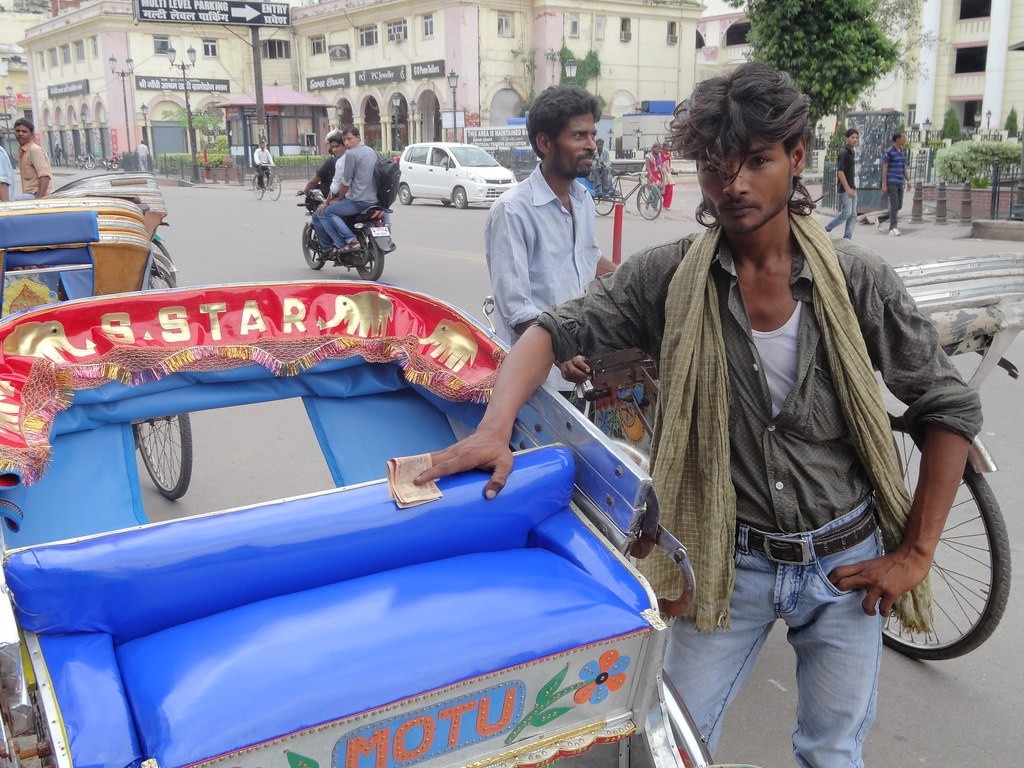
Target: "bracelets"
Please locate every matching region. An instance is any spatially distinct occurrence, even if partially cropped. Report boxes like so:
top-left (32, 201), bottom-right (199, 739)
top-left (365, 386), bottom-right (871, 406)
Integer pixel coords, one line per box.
top-left (907, 182), bottom-right (911, 184)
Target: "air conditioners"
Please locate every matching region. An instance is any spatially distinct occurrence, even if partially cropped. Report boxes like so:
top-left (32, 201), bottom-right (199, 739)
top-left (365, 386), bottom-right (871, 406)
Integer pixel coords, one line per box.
top-left (390, 32), bottom-right (403, 41)
top-left (620, 30), bottom-right (631, 41)
top-left (667, 34), bottom-right (678, 46)
top-left (300, 133), bottom-right (316, 146)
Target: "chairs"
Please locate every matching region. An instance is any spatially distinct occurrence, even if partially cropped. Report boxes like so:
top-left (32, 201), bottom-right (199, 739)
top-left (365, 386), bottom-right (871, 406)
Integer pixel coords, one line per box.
top-left (433, 151), bottom-right (446, 166)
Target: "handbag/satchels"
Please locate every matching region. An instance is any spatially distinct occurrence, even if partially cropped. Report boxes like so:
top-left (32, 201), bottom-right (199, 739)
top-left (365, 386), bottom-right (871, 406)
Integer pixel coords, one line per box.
top-left (372, 146), bottom-right (402, 209)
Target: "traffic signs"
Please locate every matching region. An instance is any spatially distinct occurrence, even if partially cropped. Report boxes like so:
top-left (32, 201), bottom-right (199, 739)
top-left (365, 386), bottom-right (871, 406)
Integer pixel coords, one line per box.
top-left (132, 0), bottom-right (292, 28)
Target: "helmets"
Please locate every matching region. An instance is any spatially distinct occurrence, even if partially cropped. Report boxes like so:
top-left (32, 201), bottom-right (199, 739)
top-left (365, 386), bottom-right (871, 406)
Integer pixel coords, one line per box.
top-left (328, 129), bottom-right (343, 143)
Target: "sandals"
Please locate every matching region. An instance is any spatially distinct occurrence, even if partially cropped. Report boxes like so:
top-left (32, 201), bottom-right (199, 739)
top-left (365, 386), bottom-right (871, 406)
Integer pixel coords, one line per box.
top-left (338, 240), bottom-right (361, 253)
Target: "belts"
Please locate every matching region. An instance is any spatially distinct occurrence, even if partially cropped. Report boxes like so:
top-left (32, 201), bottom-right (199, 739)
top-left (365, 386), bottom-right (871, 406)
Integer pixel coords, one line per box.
top-left (735, 505), bottom-right (878, 564)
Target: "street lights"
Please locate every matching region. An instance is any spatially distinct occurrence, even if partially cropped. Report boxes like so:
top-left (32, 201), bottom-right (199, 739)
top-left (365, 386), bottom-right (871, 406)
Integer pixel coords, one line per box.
top-left (0, 86), bottom-right (16, 169)
top-left (564, 58), bottom-right (577, 86)
top-left (109, 55), bottom-right (134, 167)
top-left (166, 43), bottom-right (200, 181)
top-left (392, 92), bottom-right (401, 151)
top-left (447, 69), bottom-right (458, 142)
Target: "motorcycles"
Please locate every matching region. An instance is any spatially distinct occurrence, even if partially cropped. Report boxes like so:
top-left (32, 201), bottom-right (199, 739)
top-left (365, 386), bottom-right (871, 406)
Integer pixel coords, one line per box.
top-left (295, 184), bottom-right (396, 281)
top-left (75, 152), bottom-right (95, 170)
top-left (103, 152), bottom-right (120, 170)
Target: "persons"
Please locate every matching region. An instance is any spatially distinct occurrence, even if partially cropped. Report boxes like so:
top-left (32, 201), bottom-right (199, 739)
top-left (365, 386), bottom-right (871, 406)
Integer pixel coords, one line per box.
top-left (876, 132), bottom-right (911, 236)
top-left (136, 140), bottom-right (150, 170)
top-left (413, 62), bottom-right (983, 768)
top-left (824, 128), bottom-right (860, 241)
top-left (645, 141), bottom-right (675, 211)
top-left (591, 138), bottom-right (614, 196)
top-left (14, 119), bottom-right (53, 199)
top-left (0, 145), bottom-right (16, 202)
top-left (302, 124), bottom-right (390, 253)
top-left (254, 139), bottom-right (275, 192)
top-left (55, 144), bottom-right (61, 165)
top-left (484, 83), bottom-right (622, 402)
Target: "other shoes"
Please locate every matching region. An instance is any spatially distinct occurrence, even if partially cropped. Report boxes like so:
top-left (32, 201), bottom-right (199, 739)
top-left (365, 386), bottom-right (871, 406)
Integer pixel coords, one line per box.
top-left (874, 217), bottom-right (882, 233)
top-left (888, 228), bottom-right (902, 236)
top-left (316, 249), bottom-right (333, 261)
top-left (327, 244), bottom-right (340, 259)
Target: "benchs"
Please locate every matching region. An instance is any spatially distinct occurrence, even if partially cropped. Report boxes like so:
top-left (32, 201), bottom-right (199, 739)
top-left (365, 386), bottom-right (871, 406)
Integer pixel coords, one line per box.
top-left (3, 441), bottom-right (652, 768)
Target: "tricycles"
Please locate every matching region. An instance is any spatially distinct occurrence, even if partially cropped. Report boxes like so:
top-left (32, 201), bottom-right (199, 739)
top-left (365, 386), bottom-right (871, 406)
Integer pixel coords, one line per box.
top-left (0, 195), bottom-right (193, 499)
top-left (575, 169), bottom-right (662, 220)
top-left (39, 172), bottom-right (169, 226)
top-left (479, 252), bottom-right (1024, 661)
top-left (0, 276), bottom-right (757, 767)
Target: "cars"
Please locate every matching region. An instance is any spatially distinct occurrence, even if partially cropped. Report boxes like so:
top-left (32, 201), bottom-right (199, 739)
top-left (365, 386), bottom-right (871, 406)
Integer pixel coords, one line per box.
top-left (398, 142), bottom-right (517, 209)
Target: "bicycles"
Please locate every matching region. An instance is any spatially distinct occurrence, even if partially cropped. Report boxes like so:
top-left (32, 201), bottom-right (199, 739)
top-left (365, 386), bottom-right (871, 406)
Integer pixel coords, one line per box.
top-left (253, 162), bottom-right (281, 201)
